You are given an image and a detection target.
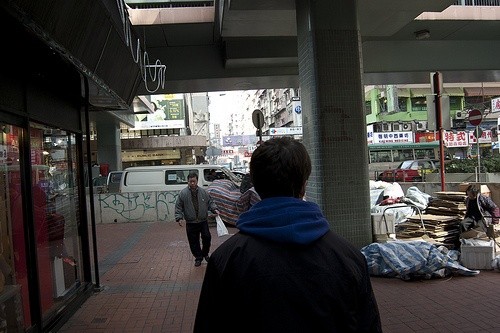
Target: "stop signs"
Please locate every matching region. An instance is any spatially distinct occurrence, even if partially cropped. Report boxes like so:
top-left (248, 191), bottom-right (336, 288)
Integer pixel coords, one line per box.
top-left (468, 109), bottom-right (482, 126)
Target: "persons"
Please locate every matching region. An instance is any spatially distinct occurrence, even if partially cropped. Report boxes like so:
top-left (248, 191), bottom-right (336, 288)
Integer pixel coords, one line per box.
top-left (462, 185), bottom-right (500, 233)
top-left (208, 171), bottom-right (218, 182)
top-left (193, 137), bottom-right (383, 333)
top-left (239, 172), bottom-right (253, 194)
top-left (175, 173), bottom-right (219, 266)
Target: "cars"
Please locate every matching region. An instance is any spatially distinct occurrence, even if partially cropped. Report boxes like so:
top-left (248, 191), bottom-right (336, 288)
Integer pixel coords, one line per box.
top-left (215, 170), bottom-right (246, 181)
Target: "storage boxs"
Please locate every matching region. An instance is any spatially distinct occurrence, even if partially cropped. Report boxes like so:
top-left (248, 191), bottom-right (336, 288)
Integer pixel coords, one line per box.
top-left (370, 212), bottom-right (394, 235)
top-left (461, 240), bottom-right (495, 269)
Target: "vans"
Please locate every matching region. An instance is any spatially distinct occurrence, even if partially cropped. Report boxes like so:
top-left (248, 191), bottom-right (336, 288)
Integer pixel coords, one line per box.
top-left (119, 165), bottom-right (242, 193)
top-left (107, 171), bottom-right (123, 193)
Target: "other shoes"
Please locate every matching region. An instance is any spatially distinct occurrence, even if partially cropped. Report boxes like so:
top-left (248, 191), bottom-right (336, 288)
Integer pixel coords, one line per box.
top-left (195, 258), bottom-right (203, 266)
top-left (204, 256), bottom-right (210, 262)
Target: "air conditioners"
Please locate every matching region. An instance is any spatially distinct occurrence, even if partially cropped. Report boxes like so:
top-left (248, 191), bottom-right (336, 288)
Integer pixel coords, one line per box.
top-left (455, 111), bottom-right (465, 119)
top-left (416, 122), bottom-right (426, 131)
top-left (382, 122), bottom-right (411, 132)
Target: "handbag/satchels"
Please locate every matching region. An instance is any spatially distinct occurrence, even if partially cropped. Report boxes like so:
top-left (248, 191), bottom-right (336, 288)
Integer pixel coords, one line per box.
top-left (215, 216), bottom-right (228, 237)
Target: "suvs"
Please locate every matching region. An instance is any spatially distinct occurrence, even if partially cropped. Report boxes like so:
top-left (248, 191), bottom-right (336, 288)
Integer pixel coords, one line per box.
top-left (381, 159), bottom-right (440, 182)
